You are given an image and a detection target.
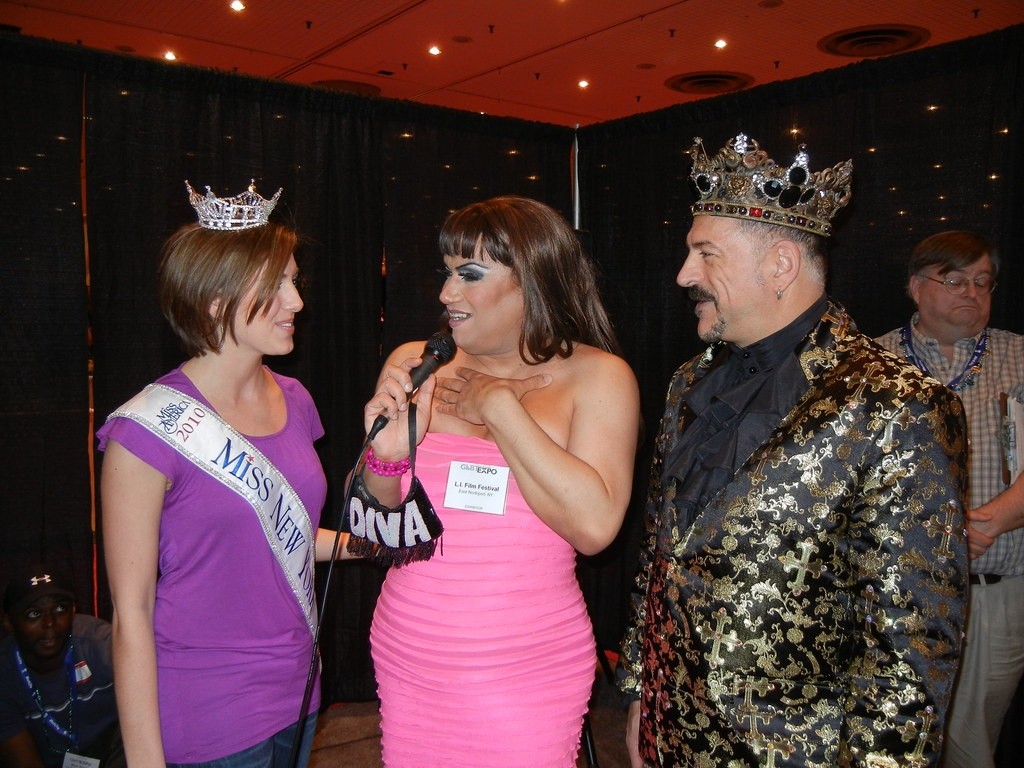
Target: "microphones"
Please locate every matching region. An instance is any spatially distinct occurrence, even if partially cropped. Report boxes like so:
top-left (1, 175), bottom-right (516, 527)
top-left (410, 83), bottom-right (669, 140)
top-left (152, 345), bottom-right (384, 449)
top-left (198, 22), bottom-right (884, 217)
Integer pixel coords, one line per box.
top-left (369, 331), bottom-right (457, 440)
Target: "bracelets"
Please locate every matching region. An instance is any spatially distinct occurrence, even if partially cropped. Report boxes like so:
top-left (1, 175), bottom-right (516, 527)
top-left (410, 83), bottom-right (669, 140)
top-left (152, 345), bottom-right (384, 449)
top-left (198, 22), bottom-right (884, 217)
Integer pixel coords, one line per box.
top-left (364, 449), bottom-right (413, 477)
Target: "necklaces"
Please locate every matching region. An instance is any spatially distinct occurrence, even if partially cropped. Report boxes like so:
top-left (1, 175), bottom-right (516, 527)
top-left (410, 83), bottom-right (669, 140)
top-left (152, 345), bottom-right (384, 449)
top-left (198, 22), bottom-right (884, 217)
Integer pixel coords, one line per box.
top-left (900, 319), bottom-right (991, 391)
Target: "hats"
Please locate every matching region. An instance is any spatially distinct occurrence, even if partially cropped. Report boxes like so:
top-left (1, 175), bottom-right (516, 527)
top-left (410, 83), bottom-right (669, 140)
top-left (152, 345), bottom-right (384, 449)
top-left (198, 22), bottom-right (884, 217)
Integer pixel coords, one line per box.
top-left (3, 564), bottom-right (82, 618)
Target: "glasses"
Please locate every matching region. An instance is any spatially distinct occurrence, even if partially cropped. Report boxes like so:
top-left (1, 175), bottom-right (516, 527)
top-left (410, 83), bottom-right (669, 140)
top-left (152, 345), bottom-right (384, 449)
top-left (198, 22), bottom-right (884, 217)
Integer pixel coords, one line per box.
top-left (913, 273), bottom-right (1000, 296)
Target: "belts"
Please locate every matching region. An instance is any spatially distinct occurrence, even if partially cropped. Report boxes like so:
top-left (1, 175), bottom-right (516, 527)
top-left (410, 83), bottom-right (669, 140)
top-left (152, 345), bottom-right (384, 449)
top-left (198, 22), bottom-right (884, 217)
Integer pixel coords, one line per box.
top-left (970, 574), bottom-right (1001, 585)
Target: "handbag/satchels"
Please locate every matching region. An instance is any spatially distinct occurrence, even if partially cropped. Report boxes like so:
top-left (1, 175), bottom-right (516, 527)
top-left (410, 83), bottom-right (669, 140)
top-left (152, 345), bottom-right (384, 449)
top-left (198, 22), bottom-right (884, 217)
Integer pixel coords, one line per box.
top-left (346, 402), bottom-right (445, 569)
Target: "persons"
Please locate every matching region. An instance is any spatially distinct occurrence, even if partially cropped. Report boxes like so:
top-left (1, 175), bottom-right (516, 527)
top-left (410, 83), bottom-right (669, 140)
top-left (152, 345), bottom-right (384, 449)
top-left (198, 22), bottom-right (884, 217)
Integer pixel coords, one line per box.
top-left (95, 179), bottom-right (383, 768)
top-left (870, 229), bottom-right (1024, 768)
top-left (344, 198), bottom-right (639, 768)
top-left (617, 133), bottom-right (969, 768)
top-left (0, 556), bottom-right (126, 768)
